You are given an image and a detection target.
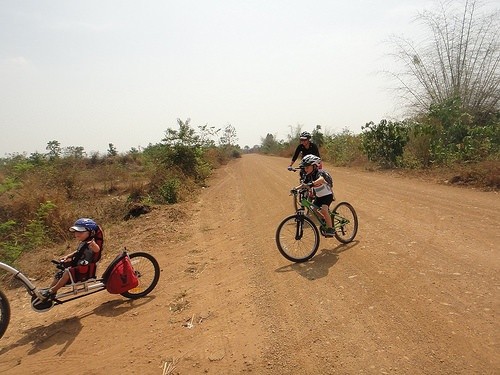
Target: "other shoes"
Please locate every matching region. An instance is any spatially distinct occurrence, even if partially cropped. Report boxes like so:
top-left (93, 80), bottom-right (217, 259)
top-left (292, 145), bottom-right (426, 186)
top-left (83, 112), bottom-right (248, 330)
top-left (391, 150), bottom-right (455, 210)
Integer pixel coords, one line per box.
top-left (39, 288), bottom-right (57, 296)
top-left (325, 227), bottom-right (336, 235)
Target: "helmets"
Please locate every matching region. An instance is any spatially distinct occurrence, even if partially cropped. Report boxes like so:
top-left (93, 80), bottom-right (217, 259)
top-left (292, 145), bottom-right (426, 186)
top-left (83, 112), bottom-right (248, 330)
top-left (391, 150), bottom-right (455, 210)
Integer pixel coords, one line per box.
top-left (299, 154), bottom-right (321, 167)
top-left (69, 218), bottom-right (98, 232)
top-left (299, 132), bottom-right (311, 140)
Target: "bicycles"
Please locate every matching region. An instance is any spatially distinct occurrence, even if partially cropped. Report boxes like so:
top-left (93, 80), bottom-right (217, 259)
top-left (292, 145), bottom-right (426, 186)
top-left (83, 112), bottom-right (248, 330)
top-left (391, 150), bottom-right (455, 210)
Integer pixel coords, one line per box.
top-left (275, 184), bottom-right (358, 263)
top-left (287, 166), bottom-right (329, 220)
top-left (0, 231), bottom-right (160, 338)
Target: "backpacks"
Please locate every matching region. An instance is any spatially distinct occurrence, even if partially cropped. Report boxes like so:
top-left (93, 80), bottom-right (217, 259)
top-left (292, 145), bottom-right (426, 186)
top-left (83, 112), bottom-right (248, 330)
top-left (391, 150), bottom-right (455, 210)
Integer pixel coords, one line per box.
top-left (315, 171), bottom-right (332, 187)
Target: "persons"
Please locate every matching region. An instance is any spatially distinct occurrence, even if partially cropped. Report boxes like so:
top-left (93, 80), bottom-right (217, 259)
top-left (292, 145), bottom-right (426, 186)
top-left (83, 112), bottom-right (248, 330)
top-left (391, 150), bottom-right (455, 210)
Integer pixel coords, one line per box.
top-left (33, 218), bottom-right (100, 302)
top-left (287, 131), bottom-right (336, 236)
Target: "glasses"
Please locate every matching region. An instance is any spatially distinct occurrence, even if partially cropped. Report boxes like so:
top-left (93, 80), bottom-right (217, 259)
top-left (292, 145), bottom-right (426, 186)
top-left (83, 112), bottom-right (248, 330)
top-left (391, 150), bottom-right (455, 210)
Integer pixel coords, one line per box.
top-left (300, 139), bottom-right (307, 141)
